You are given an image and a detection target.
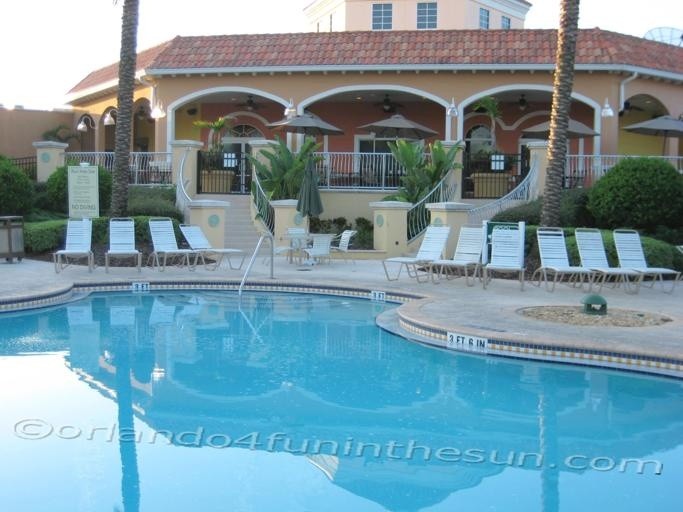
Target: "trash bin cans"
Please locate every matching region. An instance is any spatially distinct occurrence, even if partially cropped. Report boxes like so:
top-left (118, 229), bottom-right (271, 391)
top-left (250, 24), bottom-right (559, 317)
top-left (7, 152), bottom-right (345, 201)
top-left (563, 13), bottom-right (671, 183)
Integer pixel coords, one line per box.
top-left (0, 216), bottom-right (24, 262)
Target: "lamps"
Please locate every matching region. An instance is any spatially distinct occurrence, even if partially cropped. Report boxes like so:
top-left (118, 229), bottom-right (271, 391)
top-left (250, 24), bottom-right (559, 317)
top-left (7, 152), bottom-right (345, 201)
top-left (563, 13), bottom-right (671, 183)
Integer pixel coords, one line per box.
top-left (75, 109), bottom-right (116, 132)
top-left (619, 102), bottom-right (635, 116)
top-left (284, 99), bottom-right (297, 120)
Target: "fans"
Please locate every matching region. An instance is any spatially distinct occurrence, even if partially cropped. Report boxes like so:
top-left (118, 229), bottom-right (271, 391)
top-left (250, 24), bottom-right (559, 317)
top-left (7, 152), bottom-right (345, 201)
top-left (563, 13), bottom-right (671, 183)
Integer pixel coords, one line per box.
top-left (370, 95), bottom-right (404, 113)
top-left (509, 95), bottom-right (536, 112)
top-left (234, 95), bottom-right (268, 112)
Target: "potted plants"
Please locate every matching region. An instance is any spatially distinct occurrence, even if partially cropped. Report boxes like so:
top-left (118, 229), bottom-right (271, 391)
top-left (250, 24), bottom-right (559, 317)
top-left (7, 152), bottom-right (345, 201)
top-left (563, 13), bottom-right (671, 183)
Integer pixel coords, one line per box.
top-left (469, 96), bottom-right (509, 198)
top-left (189, 113), bottom-right (238, 193)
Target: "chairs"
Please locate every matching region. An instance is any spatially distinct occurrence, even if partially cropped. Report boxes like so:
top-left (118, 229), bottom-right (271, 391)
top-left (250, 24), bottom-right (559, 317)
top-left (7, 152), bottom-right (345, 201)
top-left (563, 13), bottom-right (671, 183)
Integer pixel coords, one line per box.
top-left (265, 226), bottom-right (357, 268)
top-left (52, 216), bottom-right (247, 273)
top-left (382, 220), bottom-right (681, 294)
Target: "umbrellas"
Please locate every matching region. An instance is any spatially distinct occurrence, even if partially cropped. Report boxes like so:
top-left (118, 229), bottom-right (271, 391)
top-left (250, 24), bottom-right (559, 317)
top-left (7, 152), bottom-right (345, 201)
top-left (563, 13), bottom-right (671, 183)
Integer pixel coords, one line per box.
top-left (265, 115), bottom-right (344, 142)
top-left (621, 115), bottom-right (683, 155)
top-left (296, 153), bottom-right (324, 232)
top-left (521, 118), bottom-right (600, 137)
top-left (355, 115), bottom-right (439, 138)
